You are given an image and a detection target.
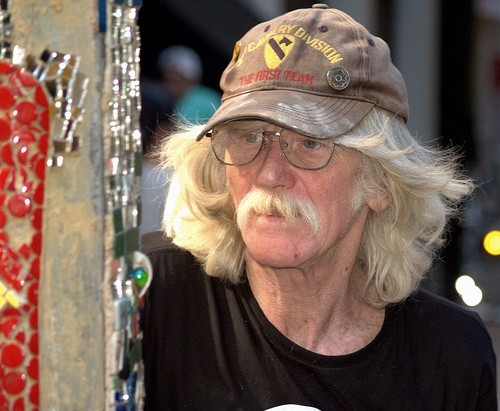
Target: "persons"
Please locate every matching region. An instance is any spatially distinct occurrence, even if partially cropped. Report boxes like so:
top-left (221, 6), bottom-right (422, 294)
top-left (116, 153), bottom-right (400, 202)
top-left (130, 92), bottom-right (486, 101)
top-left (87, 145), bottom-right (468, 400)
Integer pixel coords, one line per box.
top-left (158, 47), bottom-right (221, 127)
top-left (140, 3), bottom-right (498, 411)
top-left (140, 122), bottom-right (178, 234)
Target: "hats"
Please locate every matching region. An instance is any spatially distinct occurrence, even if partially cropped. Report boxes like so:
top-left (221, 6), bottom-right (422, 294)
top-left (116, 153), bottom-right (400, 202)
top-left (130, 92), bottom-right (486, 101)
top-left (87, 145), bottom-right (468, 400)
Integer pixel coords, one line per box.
top-left (196, 4), bottom-right (410, 143)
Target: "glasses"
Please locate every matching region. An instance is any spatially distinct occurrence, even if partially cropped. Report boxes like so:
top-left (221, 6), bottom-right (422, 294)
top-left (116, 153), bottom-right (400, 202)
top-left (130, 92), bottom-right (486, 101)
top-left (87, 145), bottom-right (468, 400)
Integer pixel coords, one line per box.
top-left (209, 122), bottom-right (339, 171)
